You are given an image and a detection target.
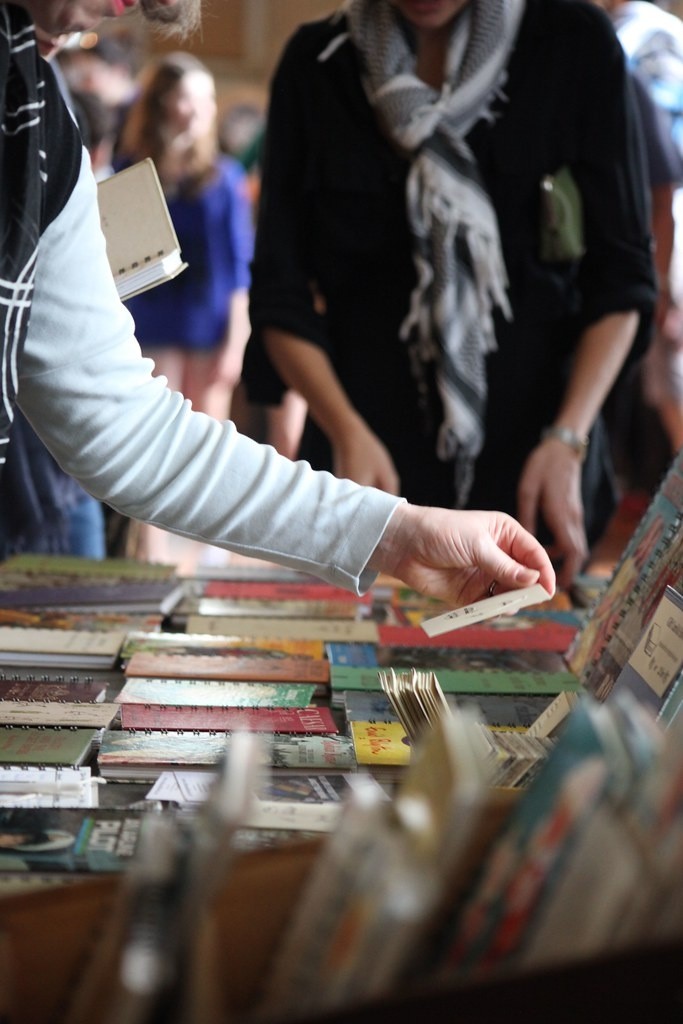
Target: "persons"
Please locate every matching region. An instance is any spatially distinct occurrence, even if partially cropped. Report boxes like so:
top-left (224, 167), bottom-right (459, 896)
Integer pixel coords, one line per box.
top-left (112, 56), bottom-right (260, 568)
top-left (38, 3), bottom-right (683, 500)
top-left (0, 2), bottom-right (562, 619)
top-left (250, 2), bottom-right (660, 593)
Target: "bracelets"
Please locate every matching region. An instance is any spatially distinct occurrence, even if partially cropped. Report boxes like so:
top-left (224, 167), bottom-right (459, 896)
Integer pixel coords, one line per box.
top-left (542, 425), bottom-right (591, 460)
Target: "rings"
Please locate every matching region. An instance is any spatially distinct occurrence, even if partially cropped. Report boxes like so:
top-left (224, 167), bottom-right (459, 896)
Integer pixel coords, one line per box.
top-left (489, 579), bottom-right (497, 595)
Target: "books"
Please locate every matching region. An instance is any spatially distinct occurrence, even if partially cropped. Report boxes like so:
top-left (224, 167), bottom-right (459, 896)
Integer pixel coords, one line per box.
top-left (96, 155), bottom-right (192, 303)
top-left (1, 541), bottom-right (683, 1024)
